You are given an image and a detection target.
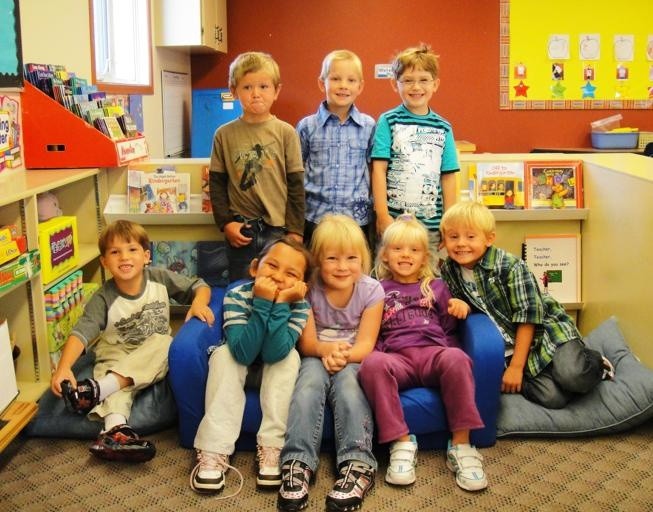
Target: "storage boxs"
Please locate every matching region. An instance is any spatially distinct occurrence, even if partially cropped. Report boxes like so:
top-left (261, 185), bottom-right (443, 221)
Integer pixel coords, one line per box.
top-left (590, 131), bottom-right (640, 151)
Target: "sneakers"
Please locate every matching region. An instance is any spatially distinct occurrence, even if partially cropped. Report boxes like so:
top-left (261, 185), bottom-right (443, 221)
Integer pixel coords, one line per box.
top-left (62, 378), bottom-right (99, 415)
top-left (194, 449), bottom-right (230, 493)
top-left (445, 439), bottom-right (488, 492)
top-left (257, 447), bottom-right (282, 487)
top-left (89, 424), bottom-right (156, 463)
top-left (277, 459), bottom-right (311, 512)
top-left (386, 434), bottom-right (419, 486)
top-left (325, 463), bottom-right (377, 510)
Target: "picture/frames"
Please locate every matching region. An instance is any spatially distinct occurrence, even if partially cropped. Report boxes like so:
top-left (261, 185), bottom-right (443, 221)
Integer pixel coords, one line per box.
top-left (523, 160), bottom-right (584, 212)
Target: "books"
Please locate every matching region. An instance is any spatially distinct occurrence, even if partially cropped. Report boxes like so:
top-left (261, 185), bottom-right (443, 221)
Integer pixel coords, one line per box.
top-left (23, 61), bottom-right (198, 214)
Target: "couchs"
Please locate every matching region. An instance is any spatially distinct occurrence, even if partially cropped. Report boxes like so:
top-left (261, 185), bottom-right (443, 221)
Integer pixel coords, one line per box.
top-left (166, 279), bottom-right (504, 450)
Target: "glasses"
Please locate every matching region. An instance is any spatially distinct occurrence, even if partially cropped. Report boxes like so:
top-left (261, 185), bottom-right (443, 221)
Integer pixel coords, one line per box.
top-left (396, 79), bottom-right (436, 89)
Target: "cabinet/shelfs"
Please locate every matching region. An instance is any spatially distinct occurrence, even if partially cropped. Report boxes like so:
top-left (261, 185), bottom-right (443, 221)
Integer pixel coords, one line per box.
top-left (104, 158), bottom-right (585, 345)
top-left (0, 164), bottom-right (109, 461)
top-left (150, 1), bottom-right (229, 53)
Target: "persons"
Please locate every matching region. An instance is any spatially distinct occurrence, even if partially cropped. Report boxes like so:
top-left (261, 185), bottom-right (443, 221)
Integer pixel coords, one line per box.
top-left (440, 199), bottom-right (614, 409)
top-left (360, 213), bottom-right (488, 491)
top-left (278, 215), bottom-right (386, 511)
top-left (190, 237), bottom-right (311, 499)
top-left (51, 218), bottom-right (215, 464)
top-left (209, 51), bottom-right (305, 285)
top-left (296, 49), bottom-right (376, 247)
top-left (370, 42), bottom-right (459, 235)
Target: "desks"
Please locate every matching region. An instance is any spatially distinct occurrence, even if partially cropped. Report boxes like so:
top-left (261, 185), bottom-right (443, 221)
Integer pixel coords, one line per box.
top-left (532, 143), bottom-right (645, 154)
